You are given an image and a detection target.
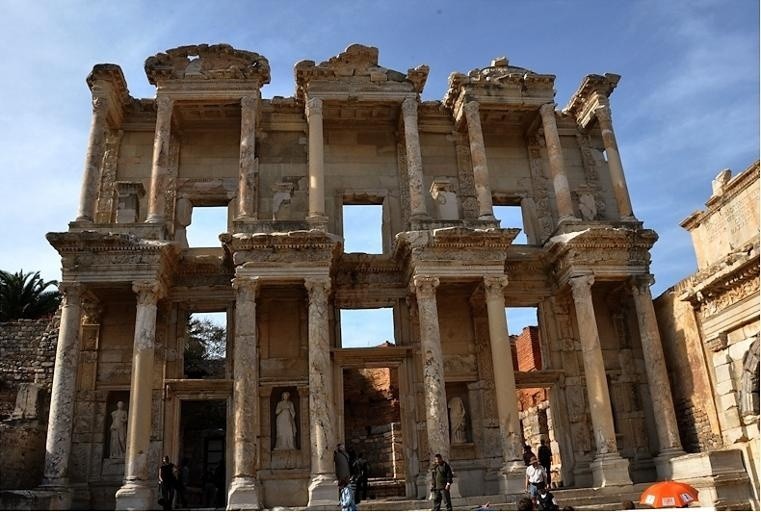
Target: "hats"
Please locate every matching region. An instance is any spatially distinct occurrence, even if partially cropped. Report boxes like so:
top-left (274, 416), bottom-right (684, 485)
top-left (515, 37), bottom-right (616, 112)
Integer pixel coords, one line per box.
top-left (529, 456), bottom-right (538, 464)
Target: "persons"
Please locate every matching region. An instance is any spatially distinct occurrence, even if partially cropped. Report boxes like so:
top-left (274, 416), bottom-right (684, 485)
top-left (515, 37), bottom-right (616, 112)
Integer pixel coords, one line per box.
top-left (518, 490), bottom-right (575, 511)
top-left (622, 500), bottom-right (636, 509)
top-left (521, 444), bottom-right (536, 466)
top-left (336, 479), bottom-right (357, 511)
top-left (538, 439), bottom-right (554, 488)
top-left (159, 455), bottom-right (179, 510)
top-left (525, 455), bottom-right (548, 507)
top-left (430, 453), bottom-right (454, 511)
top-left (110, 401), bottom-right (128, 461)
top-left (272, 390), bottom-right (297, 450)
top-left (353, 452), bottom-right (371, 498)
top-left (334, 442), bottom-right (351, 482)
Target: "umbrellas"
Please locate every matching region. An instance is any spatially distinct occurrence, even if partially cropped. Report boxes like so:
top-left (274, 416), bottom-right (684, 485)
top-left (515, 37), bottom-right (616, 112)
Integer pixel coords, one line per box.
top-left (639, 474), bottom-right (698, 509)
